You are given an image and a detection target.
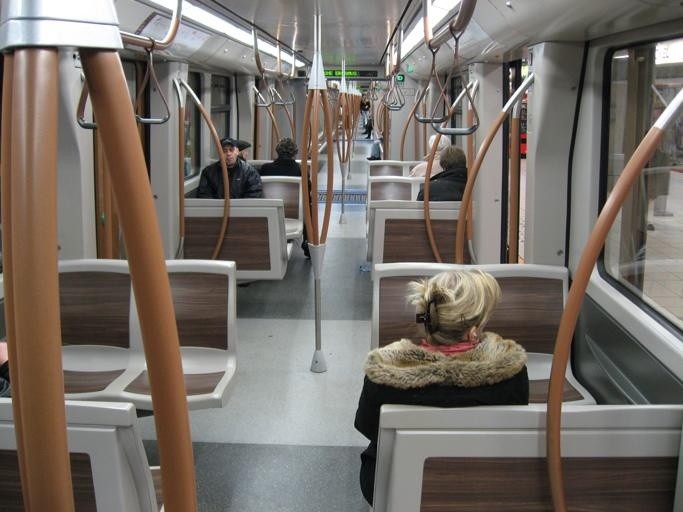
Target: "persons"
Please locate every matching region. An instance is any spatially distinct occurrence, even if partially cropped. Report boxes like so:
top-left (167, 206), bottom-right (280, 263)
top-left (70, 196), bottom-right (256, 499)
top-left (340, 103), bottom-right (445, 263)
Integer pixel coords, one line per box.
top-left (646, 103), bottom-right (676, 232)
top-left (354, 270), bottom-right (530, 507)
top-left (360, 94), bottom-right (383, 160)
top-left (235, 140), bottom-right (251, 162)
top-left (0, 341), bottom-right (13, 398)
top-left (260, 136), bottom-right (311, 259)
top-left (198, 138), bottom-right (265, 198)
top-left (409, 135), bottom-right (450, 177)
top-left (414, 145), bottom-right (467, 201)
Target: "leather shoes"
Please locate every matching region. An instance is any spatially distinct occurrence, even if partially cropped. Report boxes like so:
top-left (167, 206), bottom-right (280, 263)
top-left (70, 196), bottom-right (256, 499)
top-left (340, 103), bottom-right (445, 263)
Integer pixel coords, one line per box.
top-left (361, 132), bottom-right (370, 138)
top-left (367, 156), bottom-right (381, 160)
top-left (301, 240), bottom-right (311, 259)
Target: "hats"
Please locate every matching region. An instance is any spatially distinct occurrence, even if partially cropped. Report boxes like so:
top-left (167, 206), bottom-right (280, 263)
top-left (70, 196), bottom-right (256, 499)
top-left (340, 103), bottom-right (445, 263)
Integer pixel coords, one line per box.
top-left (234, 140), bottom-right (251, 151)
top-left (220, 138), bottom-right (237, 148)
